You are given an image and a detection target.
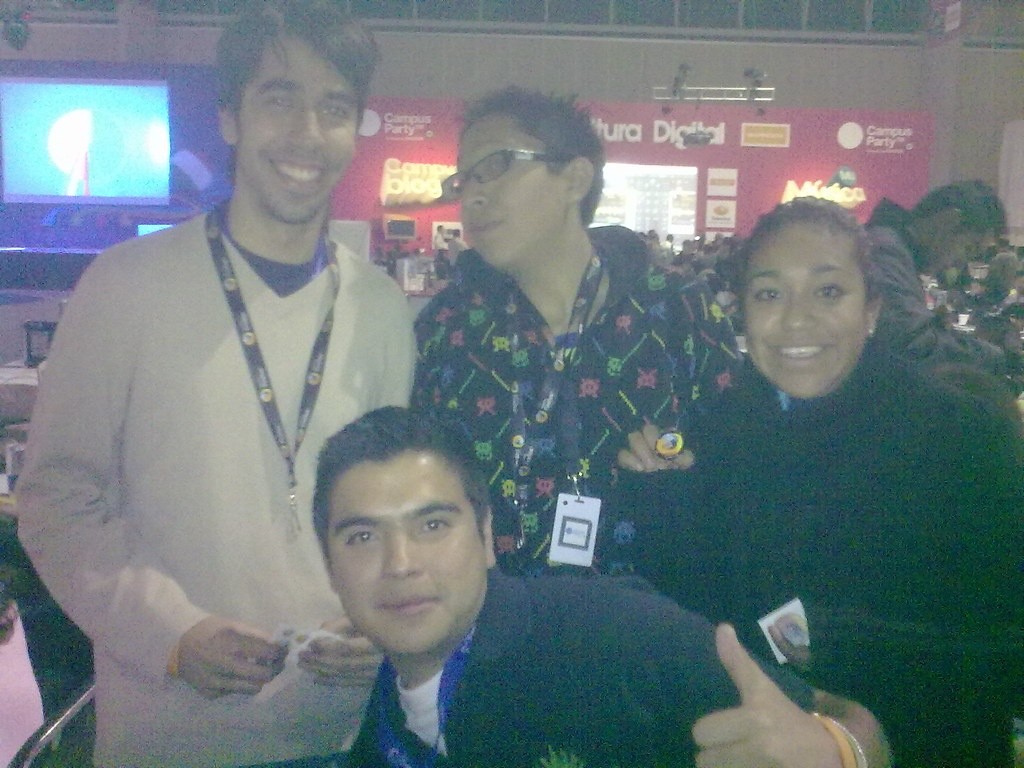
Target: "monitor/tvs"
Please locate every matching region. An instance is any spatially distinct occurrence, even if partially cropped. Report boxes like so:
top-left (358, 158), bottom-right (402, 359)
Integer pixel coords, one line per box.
top-left (0, 76), bottom-right (171, 206)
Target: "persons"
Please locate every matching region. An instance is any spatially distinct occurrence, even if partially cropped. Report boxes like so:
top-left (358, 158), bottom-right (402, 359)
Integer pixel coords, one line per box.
top-left (371, 224), bottom-right (470, 281)
top-left (617, 195), bottom-right (1024, 768)
top-left (312, 406), bottom-right (892, 768)
top-left (409, 86), bottom-right (745, 578)
top-left (936, 236), bottom-right (1024, 420)
top-left (859, 179), bottom-right (1024, 368)
top-left (639, 220), bottom-right (743, 333)
top-left (13, 0), bottom-right (417, 768)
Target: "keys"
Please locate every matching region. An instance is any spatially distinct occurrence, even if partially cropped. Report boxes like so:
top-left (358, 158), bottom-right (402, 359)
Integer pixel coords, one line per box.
top-left (288, 492), bottom-right (301, 532)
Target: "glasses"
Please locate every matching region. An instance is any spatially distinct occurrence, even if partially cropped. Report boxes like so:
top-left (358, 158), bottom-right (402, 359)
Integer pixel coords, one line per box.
top-left (441, 147), bottom-right (566, 198)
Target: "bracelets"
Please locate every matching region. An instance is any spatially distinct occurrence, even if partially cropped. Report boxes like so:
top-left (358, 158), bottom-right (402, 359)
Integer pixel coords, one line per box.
top-left (810, 710), bottom-right (868, 768)
